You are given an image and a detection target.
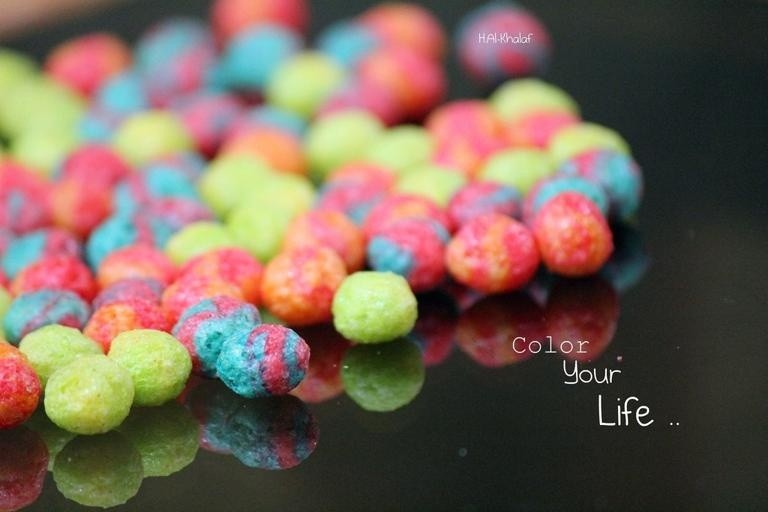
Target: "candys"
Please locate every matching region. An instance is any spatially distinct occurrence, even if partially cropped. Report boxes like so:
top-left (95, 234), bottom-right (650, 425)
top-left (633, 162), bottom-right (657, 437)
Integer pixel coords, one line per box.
top-left (0, 374), bottom-right (320, 511)
top-left (0, 0), bottom-right (646, 437)
